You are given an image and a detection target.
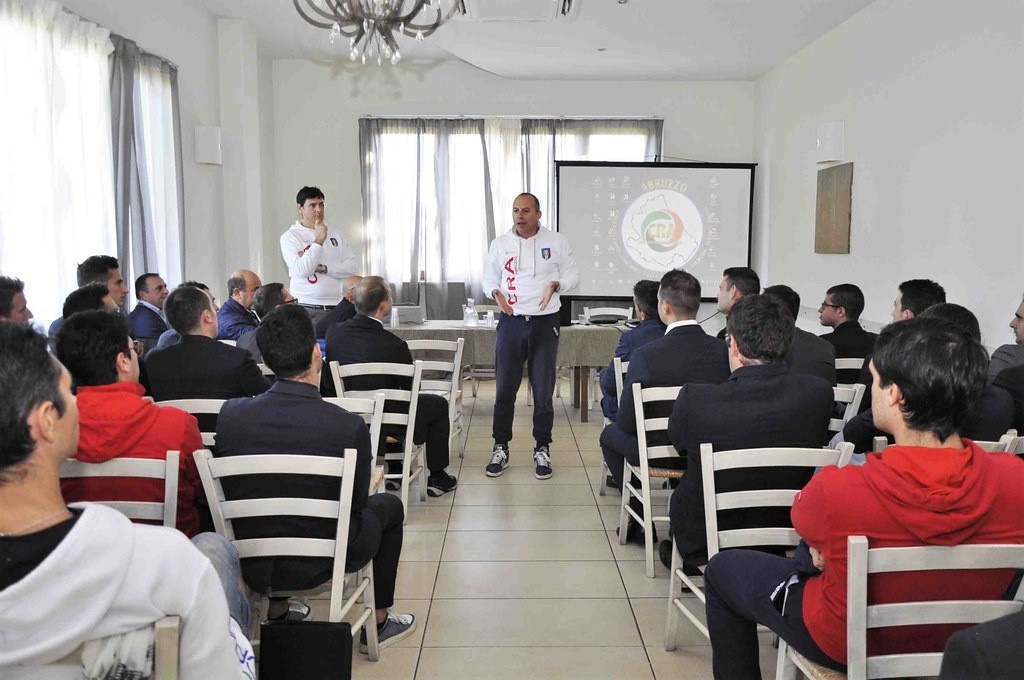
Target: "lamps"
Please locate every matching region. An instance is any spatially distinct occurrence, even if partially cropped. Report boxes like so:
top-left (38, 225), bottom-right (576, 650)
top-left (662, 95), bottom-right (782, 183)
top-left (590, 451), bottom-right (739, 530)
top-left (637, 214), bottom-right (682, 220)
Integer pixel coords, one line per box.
top-left (292, 0), bottom-right (467, 68)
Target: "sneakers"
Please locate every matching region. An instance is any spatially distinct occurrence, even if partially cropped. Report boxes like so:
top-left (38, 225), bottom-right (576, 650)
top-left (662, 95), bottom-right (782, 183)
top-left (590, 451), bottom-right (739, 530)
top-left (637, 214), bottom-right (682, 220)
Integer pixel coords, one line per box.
top-left (485, 444), bottom-right (511, 477)
top-left (427, 471), bottom-right (458, 498)
top-left (285, 602), bottom-right (314, 623)
top-left (532, 446), bottom-right (553, 480)
top-left (386, 471), bottom-right (413, 491)
top-left (359, 608), bottom-right (418, 654)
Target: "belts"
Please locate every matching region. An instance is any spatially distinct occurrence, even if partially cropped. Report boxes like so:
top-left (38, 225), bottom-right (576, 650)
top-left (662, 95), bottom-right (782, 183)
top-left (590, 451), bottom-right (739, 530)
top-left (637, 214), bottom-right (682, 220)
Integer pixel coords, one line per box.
top-left (514, 314), bottom-right (558, 321)
top-left (301, 304), bottom-right (335, 311)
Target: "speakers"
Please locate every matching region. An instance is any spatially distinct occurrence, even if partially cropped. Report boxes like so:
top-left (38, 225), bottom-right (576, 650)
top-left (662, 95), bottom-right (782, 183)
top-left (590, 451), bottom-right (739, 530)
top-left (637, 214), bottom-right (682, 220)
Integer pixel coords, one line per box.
top-left (195, 124), bottom-right (223, 164)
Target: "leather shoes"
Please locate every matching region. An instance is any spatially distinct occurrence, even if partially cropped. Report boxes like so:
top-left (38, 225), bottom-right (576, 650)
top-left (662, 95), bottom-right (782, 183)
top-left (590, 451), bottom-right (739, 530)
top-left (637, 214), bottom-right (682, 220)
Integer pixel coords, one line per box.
top-left (658, 539), bottom-right (693, 576)
top-left (616, 515), bottom-right (658, 545)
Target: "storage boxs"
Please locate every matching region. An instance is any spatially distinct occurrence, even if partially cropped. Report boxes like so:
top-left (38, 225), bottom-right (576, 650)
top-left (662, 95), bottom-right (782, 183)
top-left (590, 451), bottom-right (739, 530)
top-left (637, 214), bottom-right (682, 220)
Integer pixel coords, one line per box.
top-left (389, 306), bottom-right (425, 328)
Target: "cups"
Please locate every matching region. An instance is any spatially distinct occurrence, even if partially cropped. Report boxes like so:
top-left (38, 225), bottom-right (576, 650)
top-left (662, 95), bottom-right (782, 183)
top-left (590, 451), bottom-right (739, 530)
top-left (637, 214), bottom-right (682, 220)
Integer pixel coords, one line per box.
top-left (482, 310), bottom-right (495, 327)
top-left (579, 315), bottom-right (588, 325)
top-left (390, 308), bottom-right (399, 327)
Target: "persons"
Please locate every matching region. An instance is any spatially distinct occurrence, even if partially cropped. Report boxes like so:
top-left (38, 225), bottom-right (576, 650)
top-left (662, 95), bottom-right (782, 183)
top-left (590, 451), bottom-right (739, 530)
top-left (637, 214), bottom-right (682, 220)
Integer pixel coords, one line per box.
top-left (764, 285), bottom-right (836, 386)
top-left (600, 280), bottom-right (667, 420)
top-left (47, 255), bottom-right (328, 538)
top-left (214, 305), bottom-right (417, 653)
top-left (280, 186), bottom-right (357, 321)
top-left (716, 267), bottom-right (760, 339)
top-left (316, 275), bottom-right (363, 338)
top-left (599, 269), bottom-right (730, 544)
top-left (0, 319), bottom-right (256, 680)
top-left (939, 610), bottom-right (1024, 680)
top-left (660, 294), bottom-right (834, 592)
top-left (325, 276), bottom-right (457, 496)
top-left (819, 279), bottom-right (1024, 466)
top-left (484, 193), bottom-right (581, 479)
top-left (0, 277), bottom-right (34, 326)
top-left (704, 318), bottom-right (1024, 680)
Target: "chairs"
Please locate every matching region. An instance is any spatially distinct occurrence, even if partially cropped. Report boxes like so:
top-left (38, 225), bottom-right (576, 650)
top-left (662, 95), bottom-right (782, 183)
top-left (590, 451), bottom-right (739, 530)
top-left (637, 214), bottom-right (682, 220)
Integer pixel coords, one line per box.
top-left (557, 306), bottom-right (1024, 680)
top-left (58, 338), bottom-right (468, 680)
top-left (458, 304), bottom-right (532, 407)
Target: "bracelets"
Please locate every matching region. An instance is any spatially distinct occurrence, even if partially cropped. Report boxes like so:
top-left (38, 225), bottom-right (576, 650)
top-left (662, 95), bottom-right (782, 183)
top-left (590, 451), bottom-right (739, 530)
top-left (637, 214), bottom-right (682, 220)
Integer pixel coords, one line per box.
top-left (321, 265), bottom-right (326, 274)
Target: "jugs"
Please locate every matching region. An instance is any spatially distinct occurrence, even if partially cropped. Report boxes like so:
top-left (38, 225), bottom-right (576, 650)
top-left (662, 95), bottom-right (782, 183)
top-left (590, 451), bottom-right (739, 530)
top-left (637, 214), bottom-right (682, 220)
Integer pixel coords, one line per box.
top-left (462, 298), bottom-right (479, 327)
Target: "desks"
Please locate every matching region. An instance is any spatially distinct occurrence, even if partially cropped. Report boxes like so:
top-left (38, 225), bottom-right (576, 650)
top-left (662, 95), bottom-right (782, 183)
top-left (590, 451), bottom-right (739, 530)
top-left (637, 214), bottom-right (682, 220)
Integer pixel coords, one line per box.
top-left (383, 319), bottom-right (635, 423)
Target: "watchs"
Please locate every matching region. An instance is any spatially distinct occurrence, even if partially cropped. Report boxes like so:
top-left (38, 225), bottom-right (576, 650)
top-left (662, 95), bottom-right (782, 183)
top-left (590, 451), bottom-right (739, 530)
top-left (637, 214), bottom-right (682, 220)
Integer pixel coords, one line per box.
top-left (554, 282), bottom-right (560, 293)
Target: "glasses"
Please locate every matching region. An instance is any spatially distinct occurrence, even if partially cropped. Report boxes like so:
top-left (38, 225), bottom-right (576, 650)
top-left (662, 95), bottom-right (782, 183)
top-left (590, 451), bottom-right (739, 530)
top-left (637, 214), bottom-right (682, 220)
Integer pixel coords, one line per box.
top-left (822, 302), bottom-right (837, 311)
top-left (126, 340), bottom-right (144, 357)
top-left (285, 297), bottom-right (299, 305)
